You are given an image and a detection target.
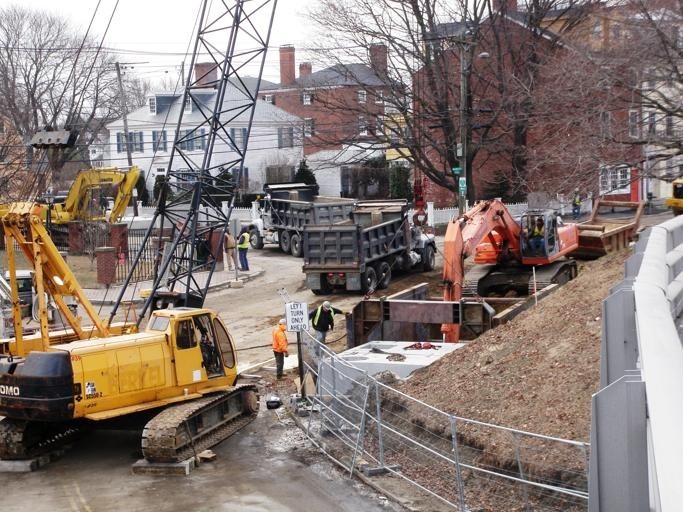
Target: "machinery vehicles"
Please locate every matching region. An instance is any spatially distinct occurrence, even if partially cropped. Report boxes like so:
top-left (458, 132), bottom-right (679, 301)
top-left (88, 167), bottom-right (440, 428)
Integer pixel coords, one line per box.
top-left (1, 166), bottom-right (142, 255)
top-left (1, 0), bottom-right (280, 477)
top-left (665, 177), bottom-right (682, 215)
top-left (439, 195), bottom-right (581, 342)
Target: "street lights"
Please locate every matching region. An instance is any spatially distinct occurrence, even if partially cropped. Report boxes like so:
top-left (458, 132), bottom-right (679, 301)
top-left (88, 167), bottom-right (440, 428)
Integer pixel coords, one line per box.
top-left (458, 51), bottom-right (490, 217)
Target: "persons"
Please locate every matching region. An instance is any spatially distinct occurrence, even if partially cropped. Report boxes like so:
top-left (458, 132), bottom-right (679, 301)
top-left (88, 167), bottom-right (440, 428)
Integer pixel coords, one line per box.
top-left (552, 211), bottom-right (563, 226)
top-left (529, 217), bottom-right (547, 256)
top-left (272, 319), bottom-right (289, 381)
top-left (98, 191), bottom-right (110, 214)
top-left (308, 300), bottom-right (350, 344)
top-left (224, 228), bottom-right (240, 271)
top-left (571, 186), bottom-right (583, 221)
top-left (238, 227), bottom-right (252, 270)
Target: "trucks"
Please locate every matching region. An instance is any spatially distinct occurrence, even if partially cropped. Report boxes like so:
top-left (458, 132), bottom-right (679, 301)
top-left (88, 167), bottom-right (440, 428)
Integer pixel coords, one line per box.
top-left (248, 180), bottom-right (360, 257)
top-left (302, 197), bottom-right (437, 294)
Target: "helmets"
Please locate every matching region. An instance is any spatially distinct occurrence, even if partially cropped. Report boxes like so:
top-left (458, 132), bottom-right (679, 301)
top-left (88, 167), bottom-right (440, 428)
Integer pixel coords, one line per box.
top-left (323, 301), bottom-right (331, 310)
top-left (279, 319), bottom-right (286, 324)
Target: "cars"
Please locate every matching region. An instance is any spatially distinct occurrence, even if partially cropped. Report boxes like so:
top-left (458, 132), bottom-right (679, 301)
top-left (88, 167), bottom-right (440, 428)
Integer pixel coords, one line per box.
top-left (1, 271), bottom-right (35, 320)
